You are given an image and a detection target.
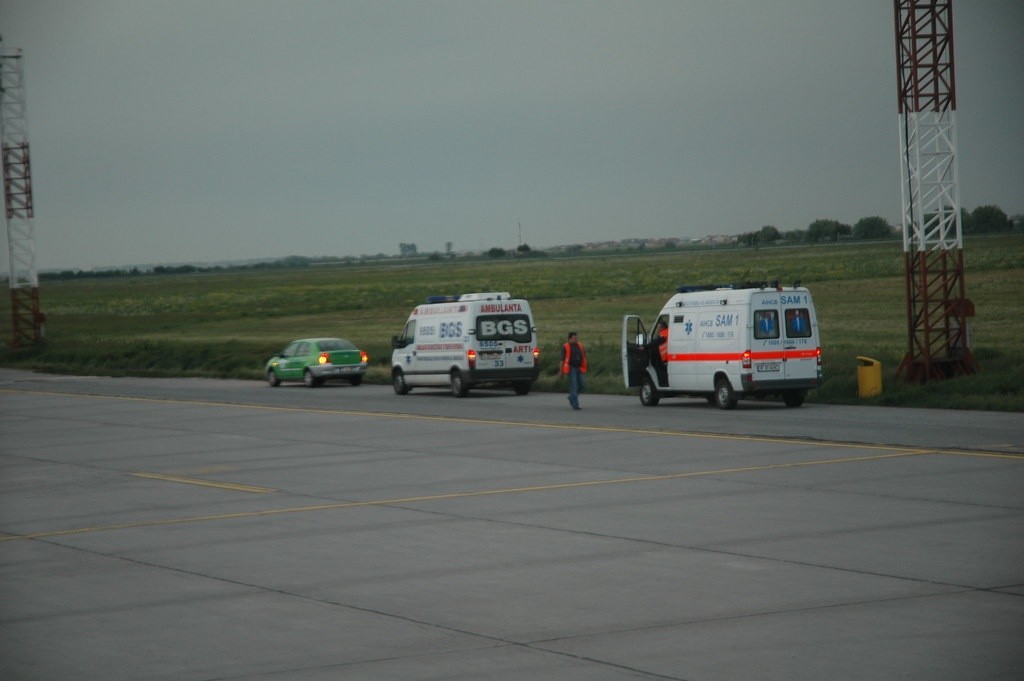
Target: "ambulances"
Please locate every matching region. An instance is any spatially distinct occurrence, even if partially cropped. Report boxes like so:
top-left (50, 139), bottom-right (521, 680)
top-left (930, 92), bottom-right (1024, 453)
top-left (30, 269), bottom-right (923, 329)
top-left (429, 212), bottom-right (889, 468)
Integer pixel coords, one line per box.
top-left (621, 281), bottom-right (823, 411)
top-left (392, 292), bottom-right (541, 398)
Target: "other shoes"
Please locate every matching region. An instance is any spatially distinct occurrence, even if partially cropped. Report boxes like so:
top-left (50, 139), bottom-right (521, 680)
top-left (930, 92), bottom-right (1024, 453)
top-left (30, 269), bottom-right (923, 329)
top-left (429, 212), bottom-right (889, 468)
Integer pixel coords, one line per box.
top-left (574, 407), bottom-right (581, 410)
top-left (567, 396), bottom-right (572, 405)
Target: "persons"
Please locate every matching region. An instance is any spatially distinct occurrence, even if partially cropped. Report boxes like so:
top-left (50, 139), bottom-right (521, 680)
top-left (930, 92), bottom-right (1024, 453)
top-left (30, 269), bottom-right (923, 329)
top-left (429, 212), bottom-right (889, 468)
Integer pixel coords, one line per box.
top-left (559, 332), bottom-right (587, 410)
top-left (638, 321), bottom-right (668, 366)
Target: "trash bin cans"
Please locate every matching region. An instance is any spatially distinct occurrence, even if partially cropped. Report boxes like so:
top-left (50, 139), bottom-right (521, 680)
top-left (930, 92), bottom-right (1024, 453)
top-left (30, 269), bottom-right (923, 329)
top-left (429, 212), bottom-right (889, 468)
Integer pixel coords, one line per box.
top-left (855, 355), bottom-right (882, 398)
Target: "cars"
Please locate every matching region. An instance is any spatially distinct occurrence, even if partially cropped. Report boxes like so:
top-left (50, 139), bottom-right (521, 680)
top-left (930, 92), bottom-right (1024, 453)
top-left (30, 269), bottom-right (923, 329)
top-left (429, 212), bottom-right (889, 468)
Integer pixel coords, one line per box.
top-left (263, 339), bottom-right (369, 389)
